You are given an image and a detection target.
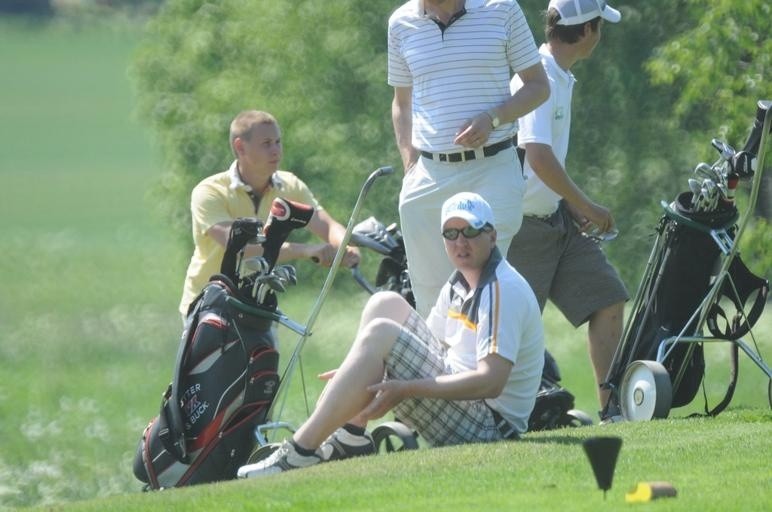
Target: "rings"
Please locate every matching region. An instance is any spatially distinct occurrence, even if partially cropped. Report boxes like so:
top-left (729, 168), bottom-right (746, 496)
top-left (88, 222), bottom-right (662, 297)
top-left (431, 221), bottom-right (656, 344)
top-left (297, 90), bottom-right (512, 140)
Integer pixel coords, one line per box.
top-left (475, 138), bottom-right (481, 147)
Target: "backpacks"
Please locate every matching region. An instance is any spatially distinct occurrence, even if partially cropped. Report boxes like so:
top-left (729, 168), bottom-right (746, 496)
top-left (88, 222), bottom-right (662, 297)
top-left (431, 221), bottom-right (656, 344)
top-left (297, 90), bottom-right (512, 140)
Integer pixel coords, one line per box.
top-left (608, 190), bottom-right (770, 411)
top-left (130, 273), bottom-right (285, 492)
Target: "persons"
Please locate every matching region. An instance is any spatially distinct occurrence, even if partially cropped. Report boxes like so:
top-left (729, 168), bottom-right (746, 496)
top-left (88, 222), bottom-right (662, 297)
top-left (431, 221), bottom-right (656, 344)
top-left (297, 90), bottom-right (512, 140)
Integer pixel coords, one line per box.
top-left (179, 108), bottom-right (360, 330)
top-left (387, 0), bottom-right (550, 324)
top-left (237, 191), bottom-right (546, 478)
top-left (505, 0), bottom-right (629, 428)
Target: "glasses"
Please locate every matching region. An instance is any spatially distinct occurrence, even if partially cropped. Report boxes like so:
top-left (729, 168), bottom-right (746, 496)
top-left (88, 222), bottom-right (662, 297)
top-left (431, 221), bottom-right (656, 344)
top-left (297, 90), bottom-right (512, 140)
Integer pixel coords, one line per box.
top-left (441, 226), bottom-right (491, 241)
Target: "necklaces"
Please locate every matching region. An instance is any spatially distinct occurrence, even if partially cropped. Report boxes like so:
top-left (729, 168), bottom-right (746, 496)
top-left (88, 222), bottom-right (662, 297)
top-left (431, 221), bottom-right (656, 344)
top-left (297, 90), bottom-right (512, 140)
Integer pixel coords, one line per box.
top-left (249, 193), bottom-right (262, 202)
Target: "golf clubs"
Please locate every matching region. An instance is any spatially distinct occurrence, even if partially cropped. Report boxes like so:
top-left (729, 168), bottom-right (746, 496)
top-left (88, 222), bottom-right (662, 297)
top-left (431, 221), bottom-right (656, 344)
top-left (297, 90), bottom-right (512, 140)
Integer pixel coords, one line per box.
top-left (242, 256), bottom-right (297, 307)
top-left (688, 138), bottom-right (736, 213)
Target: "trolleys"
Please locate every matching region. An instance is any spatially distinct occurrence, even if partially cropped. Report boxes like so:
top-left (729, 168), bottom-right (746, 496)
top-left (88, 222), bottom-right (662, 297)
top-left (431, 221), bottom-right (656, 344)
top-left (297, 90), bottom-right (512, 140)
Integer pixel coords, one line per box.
top-left (352, 215), bottom-right (592, 431)
top-left (599, 98), bottom-right (771, 423)
top-left (133, 165), bottom-right (393, 491)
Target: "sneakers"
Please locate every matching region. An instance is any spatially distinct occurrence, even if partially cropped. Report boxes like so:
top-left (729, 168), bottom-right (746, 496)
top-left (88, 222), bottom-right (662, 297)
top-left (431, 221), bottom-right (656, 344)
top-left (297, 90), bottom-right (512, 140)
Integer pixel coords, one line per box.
top-left (235, 436), bottom-right (325, 481)
top-left (320, 426), bottom-right (378, 462)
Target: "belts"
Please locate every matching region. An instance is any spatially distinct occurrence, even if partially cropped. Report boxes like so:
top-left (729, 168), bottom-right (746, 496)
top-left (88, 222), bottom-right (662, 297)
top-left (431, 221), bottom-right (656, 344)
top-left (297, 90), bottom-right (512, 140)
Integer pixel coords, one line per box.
top-left (491, 407), bottom-right (522, 442)
top-left (419, 136), bottom-right (516, 164)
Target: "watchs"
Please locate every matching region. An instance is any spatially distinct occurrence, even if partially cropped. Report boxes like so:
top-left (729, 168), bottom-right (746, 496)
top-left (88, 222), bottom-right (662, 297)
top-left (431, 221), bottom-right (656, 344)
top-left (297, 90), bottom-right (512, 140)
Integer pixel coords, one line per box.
top-left (483, 108), bottom-right (500, 130)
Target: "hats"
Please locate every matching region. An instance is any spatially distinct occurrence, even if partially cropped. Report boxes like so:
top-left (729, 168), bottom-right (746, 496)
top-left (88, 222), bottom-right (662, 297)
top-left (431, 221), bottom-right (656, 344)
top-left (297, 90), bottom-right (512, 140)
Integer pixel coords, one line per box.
top-left (439, 190), bottom-right (496, 234)
top-left (546, 0), bottom-right (623, 26)
